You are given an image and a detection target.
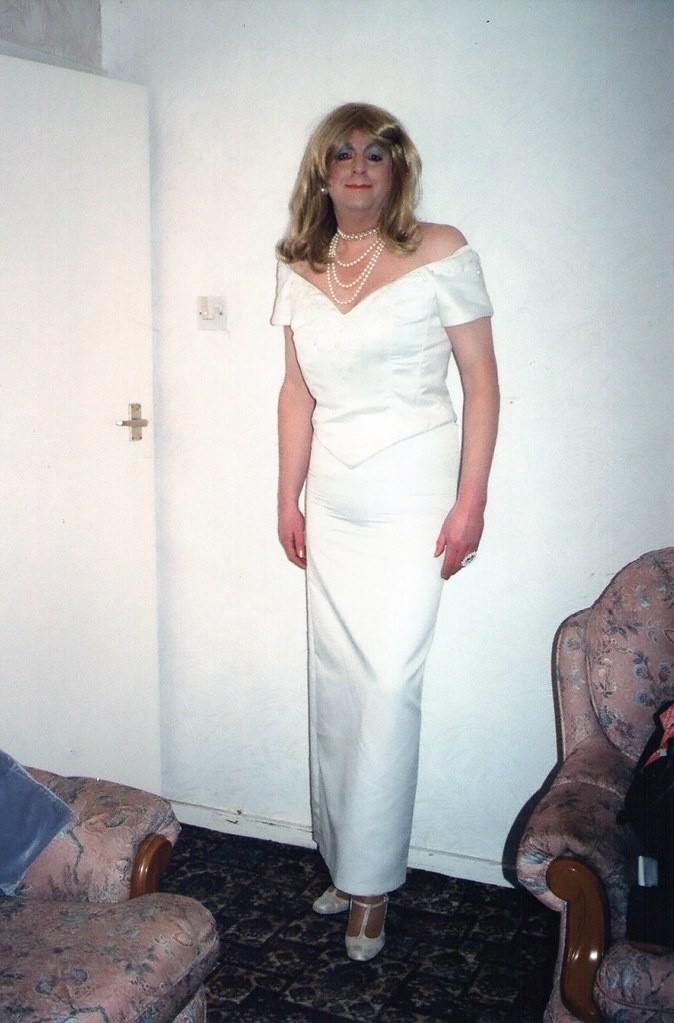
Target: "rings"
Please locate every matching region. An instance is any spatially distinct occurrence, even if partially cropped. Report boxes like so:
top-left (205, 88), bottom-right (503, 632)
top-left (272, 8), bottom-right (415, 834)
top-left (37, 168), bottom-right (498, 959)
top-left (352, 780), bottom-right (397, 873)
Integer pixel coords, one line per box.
top-left (461, 551), bottom-right (476, 567)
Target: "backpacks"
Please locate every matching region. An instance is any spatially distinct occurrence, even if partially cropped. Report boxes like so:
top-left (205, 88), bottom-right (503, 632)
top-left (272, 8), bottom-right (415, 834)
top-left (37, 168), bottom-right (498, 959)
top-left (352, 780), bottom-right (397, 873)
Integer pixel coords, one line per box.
top-left (618, 698), bottom-right (674, 956)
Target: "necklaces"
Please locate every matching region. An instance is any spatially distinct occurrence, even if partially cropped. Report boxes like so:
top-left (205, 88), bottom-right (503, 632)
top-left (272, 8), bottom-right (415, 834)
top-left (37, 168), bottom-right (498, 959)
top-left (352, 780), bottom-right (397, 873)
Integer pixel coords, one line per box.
top-left (326, 228), bottom-right (385, 306)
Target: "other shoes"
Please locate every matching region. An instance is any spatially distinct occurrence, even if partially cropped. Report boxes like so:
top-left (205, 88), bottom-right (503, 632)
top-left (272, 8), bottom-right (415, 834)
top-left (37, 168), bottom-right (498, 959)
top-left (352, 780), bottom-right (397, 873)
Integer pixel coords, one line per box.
top-left (345, 892), bottom-right (388, 962)
top-left (312, 883), bottom-right (352, 914)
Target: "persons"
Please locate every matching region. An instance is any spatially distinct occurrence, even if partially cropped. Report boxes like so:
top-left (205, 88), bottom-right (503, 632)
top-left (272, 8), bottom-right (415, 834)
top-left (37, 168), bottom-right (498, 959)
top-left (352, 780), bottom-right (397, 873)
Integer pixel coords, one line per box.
top-left (268, 101), bottom-right (501, 962)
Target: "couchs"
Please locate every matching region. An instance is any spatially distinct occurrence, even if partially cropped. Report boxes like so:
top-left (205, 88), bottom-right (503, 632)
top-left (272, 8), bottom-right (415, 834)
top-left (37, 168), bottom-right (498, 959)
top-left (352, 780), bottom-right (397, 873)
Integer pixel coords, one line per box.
top-left (514, 545), bottom-right (674, 1023)
top-left (0, 747), bottom-right (225, 1023)
